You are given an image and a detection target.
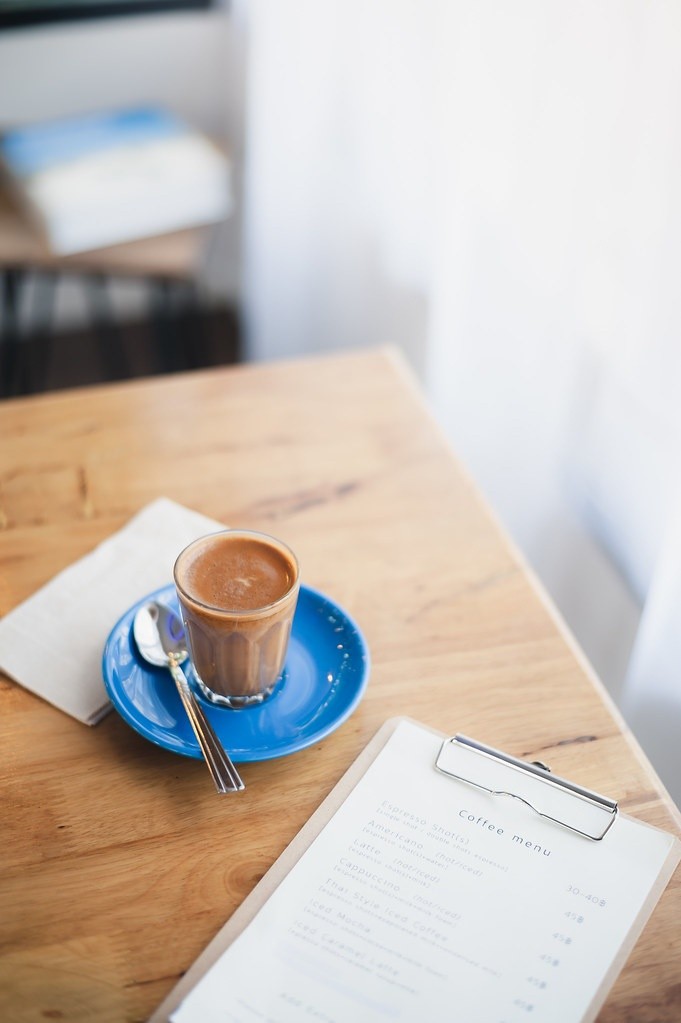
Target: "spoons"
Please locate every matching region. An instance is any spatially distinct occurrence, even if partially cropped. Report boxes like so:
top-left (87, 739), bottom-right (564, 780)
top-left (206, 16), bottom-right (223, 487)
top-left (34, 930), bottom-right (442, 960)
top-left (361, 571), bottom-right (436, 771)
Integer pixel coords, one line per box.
top-left (134, 598), bottom-right (246, 793)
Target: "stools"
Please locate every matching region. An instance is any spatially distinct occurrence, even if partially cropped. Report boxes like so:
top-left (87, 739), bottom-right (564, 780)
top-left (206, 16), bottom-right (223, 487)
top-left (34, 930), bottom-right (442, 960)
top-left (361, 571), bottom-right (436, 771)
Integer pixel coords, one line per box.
top-left (0, 134), bottom-right (229, 399)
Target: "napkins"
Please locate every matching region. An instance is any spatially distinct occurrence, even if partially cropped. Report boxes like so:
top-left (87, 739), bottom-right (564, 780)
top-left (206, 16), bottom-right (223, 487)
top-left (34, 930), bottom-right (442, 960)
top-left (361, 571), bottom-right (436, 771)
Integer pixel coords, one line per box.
top-left (0, 496), bottom-right (233, 725)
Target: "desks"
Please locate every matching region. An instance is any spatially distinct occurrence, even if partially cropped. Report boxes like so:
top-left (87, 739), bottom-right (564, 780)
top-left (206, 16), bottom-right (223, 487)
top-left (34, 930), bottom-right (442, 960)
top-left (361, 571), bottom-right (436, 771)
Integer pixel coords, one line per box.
top-left (1, 340), bottom-right (681, 1023)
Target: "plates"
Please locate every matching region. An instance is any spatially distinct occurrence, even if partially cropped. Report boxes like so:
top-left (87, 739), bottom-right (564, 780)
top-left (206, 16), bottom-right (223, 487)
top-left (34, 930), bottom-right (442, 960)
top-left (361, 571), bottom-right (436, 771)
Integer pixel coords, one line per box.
top-left (104, 582), bottom-right (368, 764)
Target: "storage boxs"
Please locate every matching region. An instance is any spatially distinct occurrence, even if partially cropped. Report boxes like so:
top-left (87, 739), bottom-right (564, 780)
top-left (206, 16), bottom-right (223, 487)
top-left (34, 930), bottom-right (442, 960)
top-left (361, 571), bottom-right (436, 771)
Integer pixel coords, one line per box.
top-left (1, 104), bottom-right (228, 256)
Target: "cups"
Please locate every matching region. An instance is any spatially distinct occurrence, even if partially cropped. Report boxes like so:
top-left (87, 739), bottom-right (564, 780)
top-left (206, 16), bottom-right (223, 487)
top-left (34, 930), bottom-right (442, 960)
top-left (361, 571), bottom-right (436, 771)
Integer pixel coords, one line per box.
top-left (174, 528), bottom-right (300, 707)
